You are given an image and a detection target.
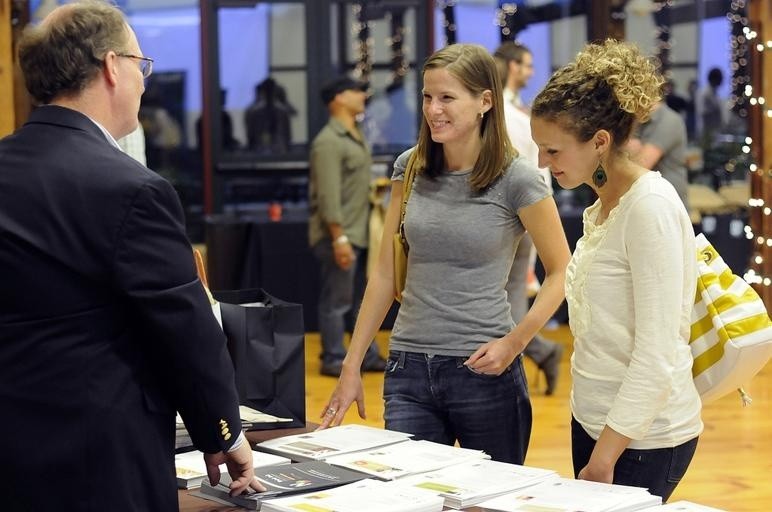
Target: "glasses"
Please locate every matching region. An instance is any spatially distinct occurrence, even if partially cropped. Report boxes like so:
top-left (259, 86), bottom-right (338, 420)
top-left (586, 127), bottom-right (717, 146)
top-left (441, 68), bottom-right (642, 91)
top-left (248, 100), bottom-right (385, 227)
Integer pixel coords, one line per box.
top-left (117, 53), bottom-right (156, 78)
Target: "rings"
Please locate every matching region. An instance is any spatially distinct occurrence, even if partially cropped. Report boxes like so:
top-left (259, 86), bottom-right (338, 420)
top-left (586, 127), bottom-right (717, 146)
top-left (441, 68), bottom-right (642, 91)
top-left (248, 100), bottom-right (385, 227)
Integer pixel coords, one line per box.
top-left (341, 258), bottom-right (346, 263)
top-left (326, 406), bottom-right (337, 415)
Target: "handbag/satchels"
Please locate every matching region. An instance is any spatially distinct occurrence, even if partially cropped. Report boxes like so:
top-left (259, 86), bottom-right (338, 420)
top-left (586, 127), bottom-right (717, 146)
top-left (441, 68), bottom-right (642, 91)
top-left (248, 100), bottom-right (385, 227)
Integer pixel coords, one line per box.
top-left (688, 230), bottom-right (772, 407)
top-left (393, 232), bottom-right (407, 304)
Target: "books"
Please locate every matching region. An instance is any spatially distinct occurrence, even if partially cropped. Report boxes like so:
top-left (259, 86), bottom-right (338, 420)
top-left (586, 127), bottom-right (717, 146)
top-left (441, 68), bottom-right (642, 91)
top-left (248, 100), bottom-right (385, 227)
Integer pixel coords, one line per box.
top-left (175, 424), bottom-right (739, 512)
top-left (173, 402), bottom-right (302, 426)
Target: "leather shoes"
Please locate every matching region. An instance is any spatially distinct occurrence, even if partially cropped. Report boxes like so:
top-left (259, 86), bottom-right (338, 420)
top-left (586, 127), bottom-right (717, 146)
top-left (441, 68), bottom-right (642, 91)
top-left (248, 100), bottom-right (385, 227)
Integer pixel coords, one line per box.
top-left (319, 353), bottom-right (388, 377)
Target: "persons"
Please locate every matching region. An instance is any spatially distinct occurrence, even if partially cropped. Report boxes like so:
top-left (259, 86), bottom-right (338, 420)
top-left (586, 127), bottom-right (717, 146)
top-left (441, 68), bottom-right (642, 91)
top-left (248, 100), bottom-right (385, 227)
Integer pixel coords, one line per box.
top-left (491, 48), bottom-right (564, 395)
top-left (658, 61), bottom-right (725, 149)
top-left (305, 75), bottom-right (388, 376)
top-left (494, 41), bottom-right (554, 283)
top-left (308, 42), bottom-right (572, 465)
top-left (0, 1), bottom-right (269, 512)
top-left (624, 99), bottom-right (688, 218)
top-left (112, 78), bottom-right (299, 178)
top-left (530, 36), bottom-right (706, 504)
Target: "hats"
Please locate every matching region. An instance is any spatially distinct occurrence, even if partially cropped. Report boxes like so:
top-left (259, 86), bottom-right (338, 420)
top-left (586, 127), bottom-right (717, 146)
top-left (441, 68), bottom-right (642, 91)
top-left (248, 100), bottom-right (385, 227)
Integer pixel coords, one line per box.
top-left (321, 79), bottom-right (369, 106)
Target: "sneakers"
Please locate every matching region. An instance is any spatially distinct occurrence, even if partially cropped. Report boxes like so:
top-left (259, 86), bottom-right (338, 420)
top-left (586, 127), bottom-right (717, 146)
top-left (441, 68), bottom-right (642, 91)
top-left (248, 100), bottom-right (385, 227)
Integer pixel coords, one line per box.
top-left (537, 342), bottom-right (563, 397)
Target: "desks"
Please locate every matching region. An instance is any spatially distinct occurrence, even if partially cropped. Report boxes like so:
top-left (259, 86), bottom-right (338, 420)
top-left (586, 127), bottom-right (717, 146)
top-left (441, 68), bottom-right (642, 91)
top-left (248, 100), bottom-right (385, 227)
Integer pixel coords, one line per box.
top-left (174, 413), bottom-right (667, 512)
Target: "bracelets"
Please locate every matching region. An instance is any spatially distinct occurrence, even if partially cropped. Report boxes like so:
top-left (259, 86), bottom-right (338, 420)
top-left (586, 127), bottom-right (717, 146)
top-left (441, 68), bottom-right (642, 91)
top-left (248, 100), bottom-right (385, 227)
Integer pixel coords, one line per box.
top-left (331, 235), bottom-right (349, 247)
